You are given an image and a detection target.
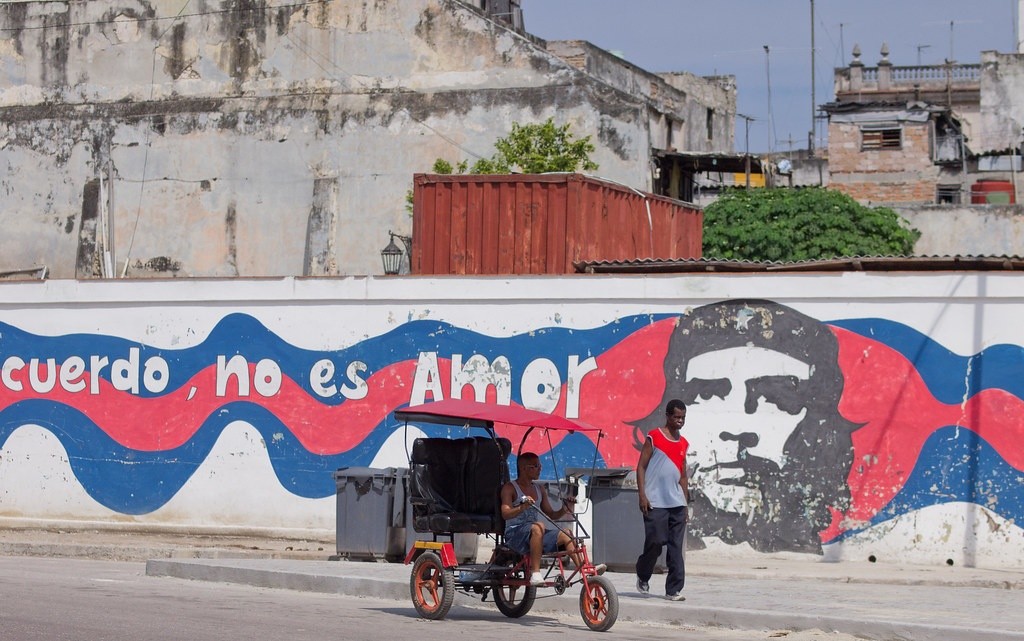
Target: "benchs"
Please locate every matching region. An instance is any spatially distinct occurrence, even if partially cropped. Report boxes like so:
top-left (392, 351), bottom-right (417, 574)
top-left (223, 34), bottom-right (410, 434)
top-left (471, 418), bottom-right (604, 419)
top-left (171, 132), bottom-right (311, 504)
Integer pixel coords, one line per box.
top-left (412, 435), bottom-right (511, 544)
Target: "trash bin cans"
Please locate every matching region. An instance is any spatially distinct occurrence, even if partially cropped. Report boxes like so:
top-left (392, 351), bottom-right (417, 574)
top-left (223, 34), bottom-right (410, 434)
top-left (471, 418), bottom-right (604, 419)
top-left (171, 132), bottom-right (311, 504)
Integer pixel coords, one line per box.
top-left (584, 468), bottom-right (688, 573)
top-left (333, 466), bottom-right (406, 560)
top-left (531, 467), bottom-right (584, 565)
top-left (405, 468), bottom-right (479, 565)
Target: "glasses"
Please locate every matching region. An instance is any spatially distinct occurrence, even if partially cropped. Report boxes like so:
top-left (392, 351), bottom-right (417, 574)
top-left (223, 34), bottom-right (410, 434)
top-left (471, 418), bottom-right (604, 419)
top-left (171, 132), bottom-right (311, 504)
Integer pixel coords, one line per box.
top-left (526, 464), bottom-right (541, 469)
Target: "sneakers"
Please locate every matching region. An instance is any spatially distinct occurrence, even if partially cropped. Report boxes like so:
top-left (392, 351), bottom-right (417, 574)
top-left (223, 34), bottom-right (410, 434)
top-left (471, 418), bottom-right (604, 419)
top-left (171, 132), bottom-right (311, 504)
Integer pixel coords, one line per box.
top-left (529, 572), bottom-right (545, 586)
top-left (636, 575), bottom-right (649, 594)
top-left (666, 591), bottom-right (685, 601)
top-left (579, 564), bottom-right (608, 584)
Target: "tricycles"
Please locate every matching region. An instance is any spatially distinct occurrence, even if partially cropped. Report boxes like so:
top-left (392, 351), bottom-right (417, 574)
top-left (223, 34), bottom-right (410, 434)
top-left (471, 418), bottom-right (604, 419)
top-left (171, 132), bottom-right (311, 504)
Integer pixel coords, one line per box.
top-left (391, 398), bottom-right (621, 632)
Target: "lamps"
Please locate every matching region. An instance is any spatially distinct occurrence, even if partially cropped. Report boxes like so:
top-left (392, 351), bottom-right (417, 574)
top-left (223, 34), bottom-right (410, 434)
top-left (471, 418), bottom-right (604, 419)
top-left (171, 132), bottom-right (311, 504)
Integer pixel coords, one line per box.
top-left (381, 230), bottom-right (412, 275)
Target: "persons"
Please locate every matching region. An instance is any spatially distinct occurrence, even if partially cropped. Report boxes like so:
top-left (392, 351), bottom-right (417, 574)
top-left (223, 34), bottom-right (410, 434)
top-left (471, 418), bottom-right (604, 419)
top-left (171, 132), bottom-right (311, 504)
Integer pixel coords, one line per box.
top-left (501, 452), bottom-right (608, 586)
top-left (635, 399), bottom-right (691, 601)
